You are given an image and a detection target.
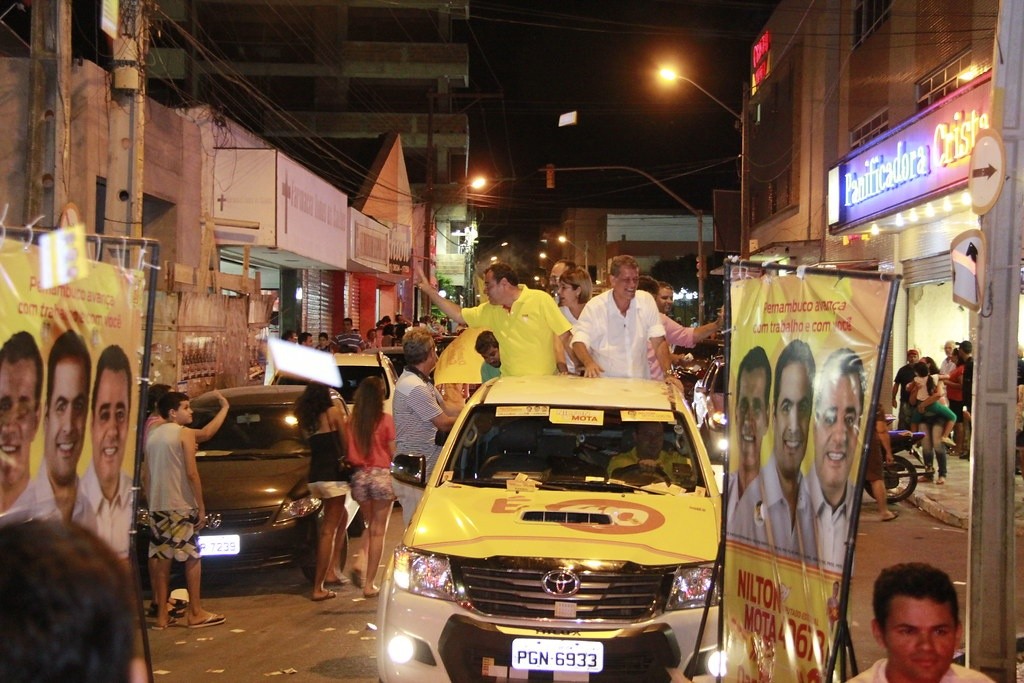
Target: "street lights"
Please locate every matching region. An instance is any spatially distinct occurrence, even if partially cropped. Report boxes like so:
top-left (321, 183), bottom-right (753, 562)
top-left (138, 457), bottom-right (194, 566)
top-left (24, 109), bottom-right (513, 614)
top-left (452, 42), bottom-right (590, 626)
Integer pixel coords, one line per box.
top-left (534, 235), bottom-right (588, 296)
top-left (656, 67), bottom-right (750, 260)
top-left (469, 241), bottom-right (508, 308)
top-left (420, 170), bottom-right (485, 319)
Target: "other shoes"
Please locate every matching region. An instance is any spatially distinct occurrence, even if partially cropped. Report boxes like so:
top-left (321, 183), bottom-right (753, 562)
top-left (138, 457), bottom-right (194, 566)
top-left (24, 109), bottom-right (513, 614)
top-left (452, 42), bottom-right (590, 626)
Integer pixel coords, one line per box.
top-left (312, 587), bottom-right (336, 600)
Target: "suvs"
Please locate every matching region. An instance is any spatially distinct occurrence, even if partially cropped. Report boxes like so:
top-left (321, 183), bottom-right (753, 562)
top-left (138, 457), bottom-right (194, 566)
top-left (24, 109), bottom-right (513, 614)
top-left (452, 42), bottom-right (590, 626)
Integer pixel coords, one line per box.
top-left (373, 373), bottom-right (725, 683)
top-left (331, 349), bottom-right (398, 418)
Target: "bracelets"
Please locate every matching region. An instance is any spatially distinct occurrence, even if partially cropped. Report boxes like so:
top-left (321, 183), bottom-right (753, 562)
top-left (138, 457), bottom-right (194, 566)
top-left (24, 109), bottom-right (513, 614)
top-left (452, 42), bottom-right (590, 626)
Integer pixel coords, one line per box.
top-left (918, 404), bottom-right (925, 408)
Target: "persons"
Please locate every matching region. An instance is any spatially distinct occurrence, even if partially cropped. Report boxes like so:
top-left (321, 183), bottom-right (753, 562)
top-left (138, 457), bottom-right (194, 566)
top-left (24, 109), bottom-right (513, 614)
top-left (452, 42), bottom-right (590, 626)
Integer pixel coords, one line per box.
top-left (0, 518), bottom-right (149, 683)
top-left (726, 340), bottom-right (866, 573)
top-left (844, 562), bottom-right (997, 683)
top-left (549, 259), bottom-right (593, 376)
top-left (892, 341), bottom-right (974, 484)
top-left (569, 255), bottom-right (684, 392)
top-left (1017, 346), bottom-right (1024, 386)
top-left (0, 329), bottom-right (134, 565)
top-left (282, 314), bottom-right (449, 354)
top-left (390, 327), bottom-right (464, 527)
top-left (293, 375), bottom-right (394, 602)
top-left (143, 384), bottom-right (229, 631)
top-left (864, 402), bottom-right (899, 522)
top-left (412, 264), bottom-right (585, 377)
top-left (637, 275), bottom-right (724, 382)
top-left (475, 331), bottom-right (501, 385)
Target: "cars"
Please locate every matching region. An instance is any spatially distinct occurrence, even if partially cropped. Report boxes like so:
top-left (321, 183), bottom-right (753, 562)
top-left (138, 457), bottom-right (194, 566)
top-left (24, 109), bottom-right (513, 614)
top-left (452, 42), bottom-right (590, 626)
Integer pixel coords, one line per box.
top-left (141, 384), bottom-right (351, 577)
top-left (692, 355), bottom-right (729, 463)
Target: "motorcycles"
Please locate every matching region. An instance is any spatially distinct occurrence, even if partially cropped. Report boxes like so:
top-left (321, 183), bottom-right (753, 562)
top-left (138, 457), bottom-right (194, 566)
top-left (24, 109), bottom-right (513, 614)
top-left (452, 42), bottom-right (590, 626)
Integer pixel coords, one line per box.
top-left (862, 430), bottom-right (934, 504)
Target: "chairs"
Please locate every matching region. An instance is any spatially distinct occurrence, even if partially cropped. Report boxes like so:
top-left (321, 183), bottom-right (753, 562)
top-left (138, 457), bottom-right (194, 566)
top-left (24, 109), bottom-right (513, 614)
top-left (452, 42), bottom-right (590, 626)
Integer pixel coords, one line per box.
top-left (479, 418), bottom-right (549, 477)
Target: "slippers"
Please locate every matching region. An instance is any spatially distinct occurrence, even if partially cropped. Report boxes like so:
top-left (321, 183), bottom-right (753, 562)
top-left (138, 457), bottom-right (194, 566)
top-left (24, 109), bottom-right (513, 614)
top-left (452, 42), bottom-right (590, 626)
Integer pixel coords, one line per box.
top-left (880, 512), bottom-right (901, 523)
top-left (323, 575), bottom-right (350, 586)
top-left (150, 614), bottom-right (181, 634)
top-left (187, 612), bottom-right (229, 629)
top-left (352, 568), bottom-right (380, 598)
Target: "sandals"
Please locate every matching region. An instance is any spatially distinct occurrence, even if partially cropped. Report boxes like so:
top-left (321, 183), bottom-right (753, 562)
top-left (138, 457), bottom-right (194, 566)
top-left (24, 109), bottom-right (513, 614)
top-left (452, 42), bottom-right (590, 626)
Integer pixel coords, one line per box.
top-left (148, 597), bottom-right (187, 618)
